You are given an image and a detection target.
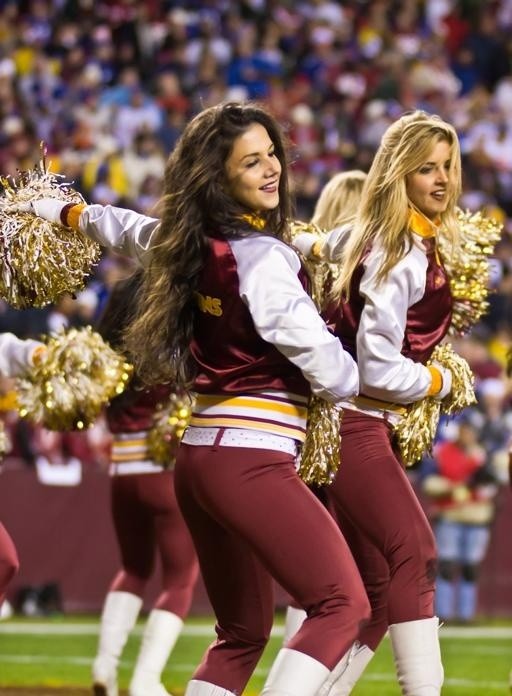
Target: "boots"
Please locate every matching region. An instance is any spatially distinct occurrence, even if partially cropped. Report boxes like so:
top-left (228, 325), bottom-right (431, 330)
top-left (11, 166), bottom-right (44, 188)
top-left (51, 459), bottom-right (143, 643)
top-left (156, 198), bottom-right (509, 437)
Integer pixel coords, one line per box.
top-left (184, 679), bottom-right (238, 696)
top-left (259, 647), bottom-right (331, 696)
top-left (128, 598), bottom-right (186, 696)
top-left (315, 639), bottom-right (376, 696)
top-left (386, 615), bottom-right (444, 696)
top-left (91, 590), bottom-right (143, 696)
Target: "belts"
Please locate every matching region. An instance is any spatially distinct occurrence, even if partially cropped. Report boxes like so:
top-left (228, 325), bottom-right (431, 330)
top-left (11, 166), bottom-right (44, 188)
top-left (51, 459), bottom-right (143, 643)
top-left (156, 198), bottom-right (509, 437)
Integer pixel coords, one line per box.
top-left (107, 460), bottom-right (169, 476)
top-left (180, 425), bottom-right (305, 457)
top-left (335, 397), bottom-right (410, 429)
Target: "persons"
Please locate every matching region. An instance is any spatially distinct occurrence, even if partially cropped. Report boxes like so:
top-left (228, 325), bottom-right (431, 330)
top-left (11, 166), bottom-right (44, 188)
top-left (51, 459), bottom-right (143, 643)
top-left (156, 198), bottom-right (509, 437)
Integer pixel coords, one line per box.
top-left (0, 1), bottom-right (511, 696)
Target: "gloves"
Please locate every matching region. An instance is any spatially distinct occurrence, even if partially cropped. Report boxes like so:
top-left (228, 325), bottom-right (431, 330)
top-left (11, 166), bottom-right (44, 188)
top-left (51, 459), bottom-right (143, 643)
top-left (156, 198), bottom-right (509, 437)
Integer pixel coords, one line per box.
top-left (7, 193), bottom-right (76, 230)
top-left (431, 358), bottom-right (452, 399)
